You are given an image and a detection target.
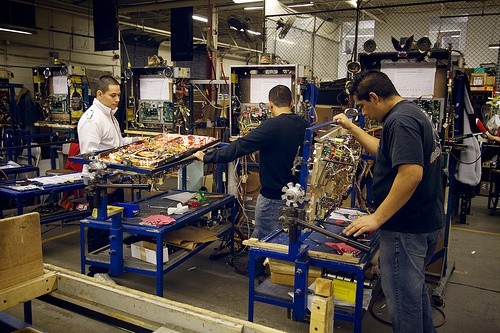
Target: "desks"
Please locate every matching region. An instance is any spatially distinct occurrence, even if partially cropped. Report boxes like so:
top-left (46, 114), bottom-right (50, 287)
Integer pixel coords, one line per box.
top-left (0, 172), bottom-right (94, 225)
top-left (0, 164), bottom-right (40, 181)
top-left (78, 190), bottom-right (236, 299)
top-left (246, 205), bottom-right (385, 333)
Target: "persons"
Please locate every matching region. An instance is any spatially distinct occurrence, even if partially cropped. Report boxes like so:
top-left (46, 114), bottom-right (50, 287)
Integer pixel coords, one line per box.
top-left (192, 84), bottom-right (311, 277)
top-left (473, 109), bottom-right (500, 141)
top-left (333, 70), bottom-right (445, 333)
top-left (77, 74), bottom-right (128, 287)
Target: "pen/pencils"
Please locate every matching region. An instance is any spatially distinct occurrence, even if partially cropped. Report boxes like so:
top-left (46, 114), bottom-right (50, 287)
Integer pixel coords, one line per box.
top-left (305, 236), bottom-right (323, 245)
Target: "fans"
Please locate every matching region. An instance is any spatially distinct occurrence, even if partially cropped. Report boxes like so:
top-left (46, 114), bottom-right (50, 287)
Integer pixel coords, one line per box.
top-left (277, 16), bottom-right (296, 40)
top-left (227, 14), bottom-right (256, 43)
